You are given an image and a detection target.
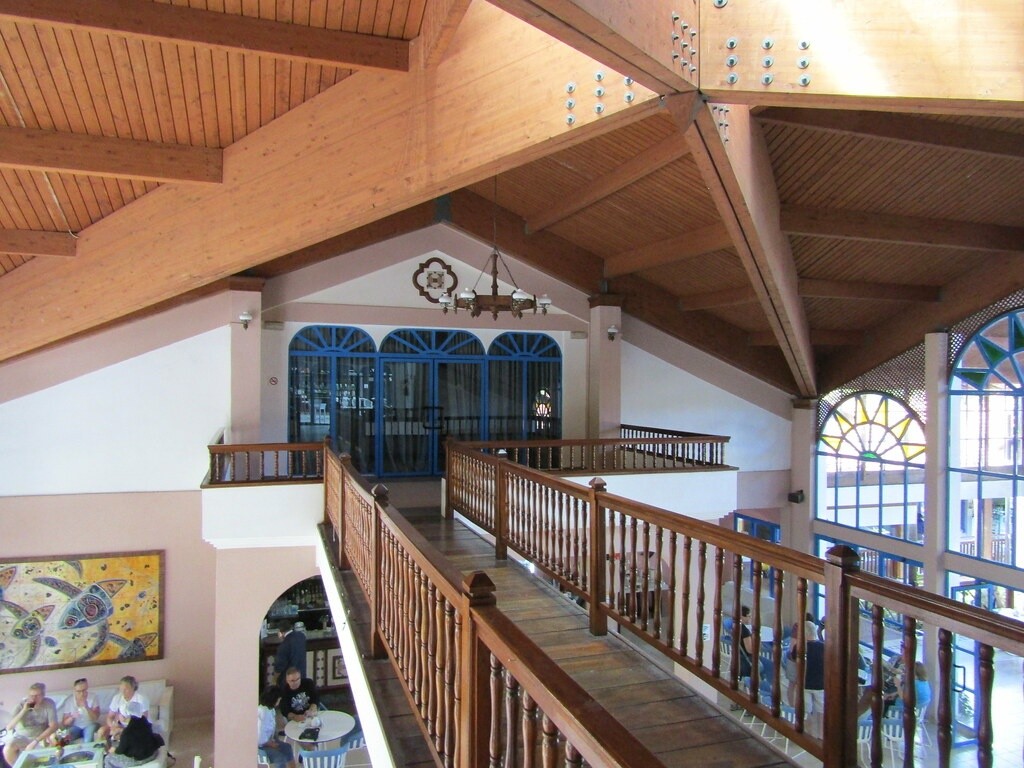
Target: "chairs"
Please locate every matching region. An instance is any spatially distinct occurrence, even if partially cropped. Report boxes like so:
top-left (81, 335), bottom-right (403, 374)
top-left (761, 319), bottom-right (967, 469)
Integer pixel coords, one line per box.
top-left (299, 741), bottom-right (351, 767)
top-left (721, 617), bottom-right (795, 723)
top-left (342, 715), bottom-right (366, 751)
top-left (857, 719), bottom-right (872, 768)
top-left (258, 751), bottom-right (270, 767)
top-left (882, 712), bottom-right (921, 768)
top-left (884, 703), bottom-right (933, 761)
top-left (283, 700), bottom-right (327, 724)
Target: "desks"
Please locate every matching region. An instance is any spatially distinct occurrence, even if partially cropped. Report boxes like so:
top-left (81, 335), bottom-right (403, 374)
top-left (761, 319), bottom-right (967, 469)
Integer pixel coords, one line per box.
top-left (281, 709), bottom-right (356, 761)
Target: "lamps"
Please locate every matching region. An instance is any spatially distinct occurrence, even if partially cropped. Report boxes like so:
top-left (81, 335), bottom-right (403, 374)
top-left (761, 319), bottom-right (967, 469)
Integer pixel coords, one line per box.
top-left (237, 309), bottom-right (253, 330)
top-left (607, 322), bottom-right (618, 340)
top-left (438, 177), bottom-right (552, 322)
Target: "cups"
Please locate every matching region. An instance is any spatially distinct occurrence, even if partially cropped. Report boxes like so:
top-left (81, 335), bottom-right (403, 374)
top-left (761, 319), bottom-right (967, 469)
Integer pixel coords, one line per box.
top-left (895, 674), bottom-right (901, 687)
top-left (111, 719), bottom-right (118, 731)
top-left (294, 622), bottom-right (305, 632)
top-left (28, 701), bottom-right (35, 712)
top-left (78, 707), bottom-right (85, 715)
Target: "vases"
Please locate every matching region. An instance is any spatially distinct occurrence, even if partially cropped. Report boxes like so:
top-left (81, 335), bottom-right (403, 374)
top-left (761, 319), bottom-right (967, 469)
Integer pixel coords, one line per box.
top-left (55, 748), bottom-right (65, 759)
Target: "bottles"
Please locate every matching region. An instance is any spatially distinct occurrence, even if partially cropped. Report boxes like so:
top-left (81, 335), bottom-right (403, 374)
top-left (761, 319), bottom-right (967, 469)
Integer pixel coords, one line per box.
top-left (269, 581), bottom-right (327, 616)
top-left (623, 559), bottom-right (640, 587)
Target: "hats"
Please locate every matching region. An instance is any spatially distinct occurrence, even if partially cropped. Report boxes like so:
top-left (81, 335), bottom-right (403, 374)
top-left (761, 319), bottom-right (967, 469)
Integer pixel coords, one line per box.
top-left (119, 702), bottom-right (143, 719)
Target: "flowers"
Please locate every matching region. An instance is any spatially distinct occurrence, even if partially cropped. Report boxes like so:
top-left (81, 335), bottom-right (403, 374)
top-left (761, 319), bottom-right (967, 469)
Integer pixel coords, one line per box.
top-left (53, 729), bottom-right (70, 745)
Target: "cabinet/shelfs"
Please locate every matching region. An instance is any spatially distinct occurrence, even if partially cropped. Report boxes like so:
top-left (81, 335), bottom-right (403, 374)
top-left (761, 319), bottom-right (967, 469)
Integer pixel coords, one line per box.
top-left (267, 577), bottom-right (333, 617)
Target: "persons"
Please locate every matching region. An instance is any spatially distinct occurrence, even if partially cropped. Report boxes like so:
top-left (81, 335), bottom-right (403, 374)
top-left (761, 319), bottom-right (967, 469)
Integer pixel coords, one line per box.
top-left (1, 678), bottom-right (163, 768)
top-left (730, 608), bottom-right (931, 736)
top-left (257, 622), bottom-right (324, 766)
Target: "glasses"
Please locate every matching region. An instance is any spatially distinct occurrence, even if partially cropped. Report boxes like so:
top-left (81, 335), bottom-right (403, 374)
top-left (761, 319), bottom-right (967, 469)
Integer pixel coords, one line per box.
top-left (75, 678), bottom-right (87, 684)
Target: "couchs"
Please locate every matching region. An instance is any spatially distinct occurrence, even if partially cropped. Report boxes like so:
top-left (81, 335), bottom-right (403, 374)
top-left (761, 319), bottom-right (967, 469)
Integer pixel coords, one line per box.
top-left (4, 676), bottom-right (176, 741)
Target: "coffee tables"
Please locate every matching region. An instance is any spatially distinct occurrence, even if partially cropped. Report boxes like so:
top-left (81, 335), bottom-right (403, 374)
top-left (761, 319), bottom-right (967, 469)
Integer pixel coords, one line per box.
top-left (13, 740), bottom-right (105, 768)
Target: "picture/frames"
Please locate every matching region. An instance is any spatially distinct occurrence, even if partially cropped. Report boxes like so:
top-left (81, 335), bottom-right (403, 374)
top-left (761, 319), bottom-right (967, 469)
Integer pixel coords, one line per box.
top-left (1, 549), bottom-right (166, 675)
